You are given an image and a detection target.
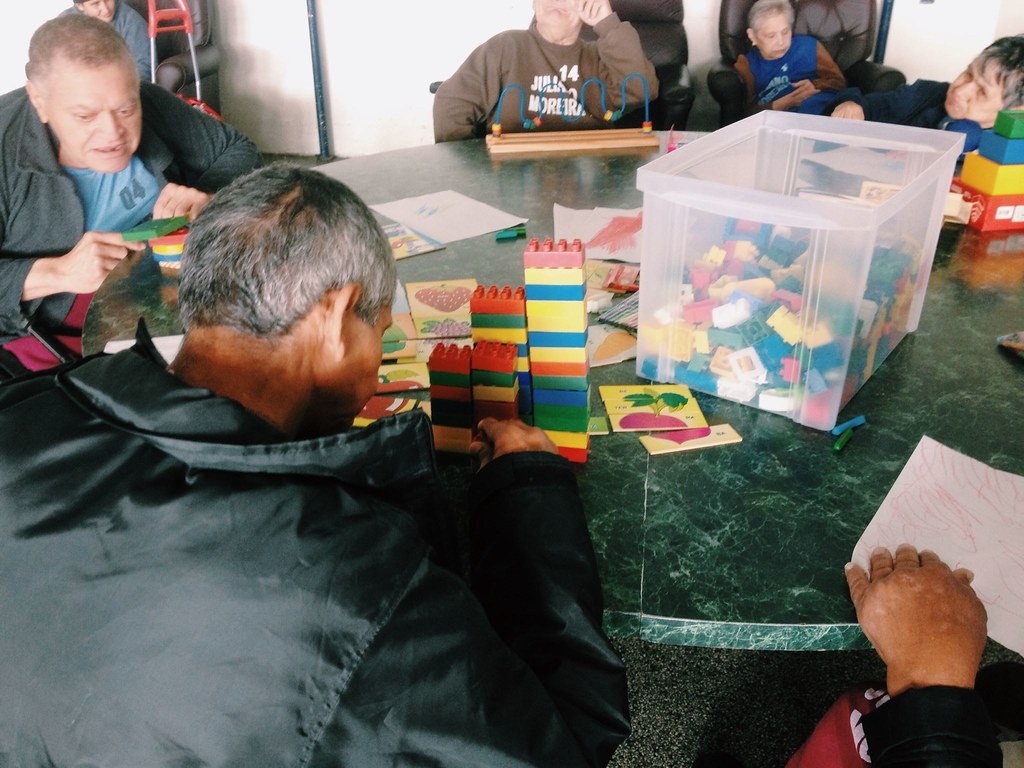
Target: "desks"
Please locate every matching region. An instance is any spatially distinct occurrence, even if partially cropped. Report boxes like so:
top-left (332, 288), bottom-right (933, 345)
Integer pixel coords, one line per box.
top-left (81, 139), bottom-right (1024, 652)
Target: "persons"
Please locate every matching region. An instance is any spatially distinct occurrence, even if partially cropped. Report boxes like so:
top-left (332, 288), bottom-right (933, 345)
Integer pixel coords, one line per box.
top-left (734, 0), bottom-right (862, 116)
top-left (58, 0), bottom-right (153, 81)
top-left (824, 34), bottom-right (1024, 169)
top-left (433, 0), bottom-right (659, 143)
top-left (0, 161), bottom-right (631, 768)
top-left (783, 543), bottom-right (1024, 768)
top-left (0, 14), bottom-right (267, 379)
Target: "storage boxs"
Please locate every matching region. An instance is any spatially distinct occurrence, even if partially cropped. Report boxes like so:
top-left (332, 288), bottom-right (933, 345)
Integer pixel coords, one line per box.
top-left (950, 110), bottom-right (1024, 232)
top-left (633, 110), bottom-right (967, 433)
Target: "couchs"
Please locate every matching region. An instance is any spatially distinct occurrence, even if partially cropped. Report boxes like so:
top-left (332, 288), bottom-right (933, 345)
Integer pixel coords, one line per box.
top-left (123, 0), bottom-right (222, 116)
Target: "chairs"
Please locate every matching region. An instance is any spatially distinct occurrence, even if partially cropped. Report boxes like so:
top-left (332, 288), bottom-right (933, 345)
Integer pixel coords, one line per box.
top-left (707, 0), bottom-right (907, 128)
top-left (430, 0), bottom-right (689, 130)
